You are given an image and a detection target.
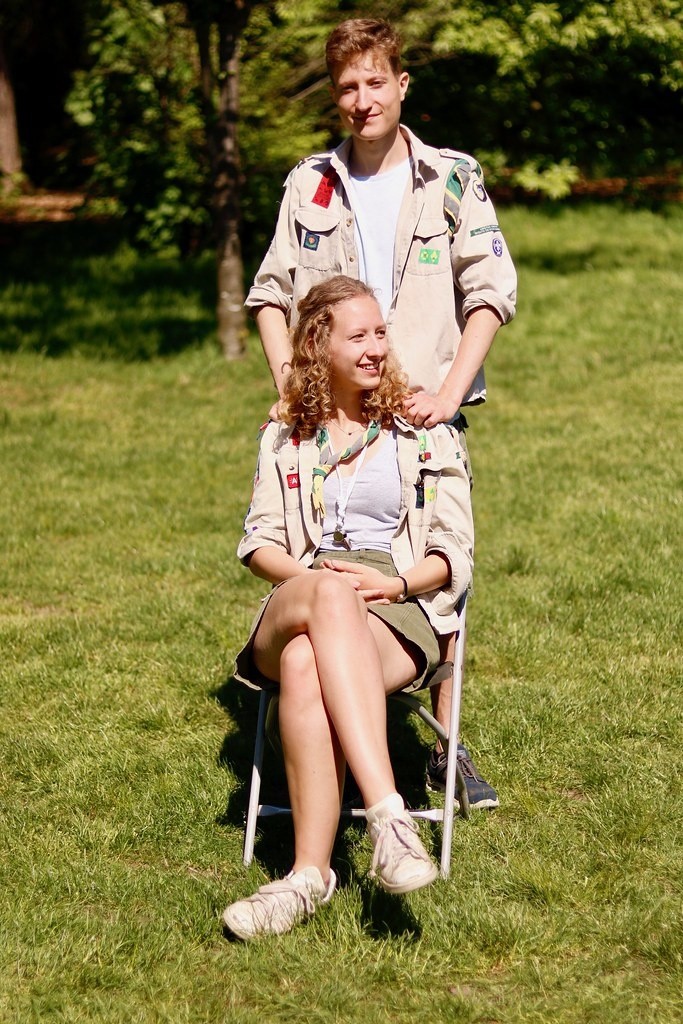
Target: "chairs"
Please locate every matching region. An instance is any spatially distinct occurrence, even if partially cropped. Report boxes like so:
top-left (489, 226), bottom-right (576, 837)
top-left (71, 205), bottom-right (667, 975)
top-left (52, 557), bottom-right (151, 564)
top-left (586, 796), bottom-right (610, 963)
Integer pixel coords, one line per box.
top-left (244, 425), bottom-right (467, 880)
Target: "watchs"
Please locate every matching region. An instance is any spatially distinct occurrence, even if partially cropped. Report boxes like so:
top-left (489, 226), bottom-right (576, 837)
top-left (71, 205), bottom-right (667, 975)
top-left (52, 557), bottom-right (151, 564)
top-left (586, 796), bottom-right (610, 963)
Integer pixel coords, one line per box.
top-left (394, 576), bottom-right (409, 603)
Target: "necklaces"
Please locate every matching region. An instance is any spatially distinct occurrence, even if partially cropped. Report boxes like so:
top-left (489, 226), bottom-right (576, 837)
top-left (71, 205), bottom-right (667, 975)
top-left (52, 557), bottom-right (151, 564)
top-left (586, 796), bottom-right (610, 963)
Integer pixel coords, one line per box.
top-left (327, 431), bottom-right (369, 542)
top-left (330, 418), bottom-right (362, 435)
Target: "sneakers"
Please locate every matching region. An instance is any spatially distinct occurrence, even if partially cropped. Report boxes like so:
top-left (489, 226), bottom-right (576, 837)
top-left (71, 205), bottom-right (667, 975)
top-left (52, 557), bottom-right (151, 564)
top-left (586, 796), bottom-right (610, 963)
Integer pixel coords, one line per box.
top-left (426, 745), bottom-right (500, 810)
top-left (224, 865), bottom-right (337, 944)
top-left (366, 813), bottom-right (438, 895)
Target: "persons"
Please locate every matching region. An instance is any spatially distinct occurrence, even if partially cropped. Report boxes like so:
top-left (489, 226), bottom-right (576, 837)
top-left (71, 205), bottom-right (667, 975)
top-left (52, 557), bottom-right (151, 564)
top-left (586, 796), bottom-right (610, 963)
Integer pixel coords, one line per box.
top-left (241, 14), bottom-right (518, 810)
top-left (223, 275), bottom-right (475, 942)
